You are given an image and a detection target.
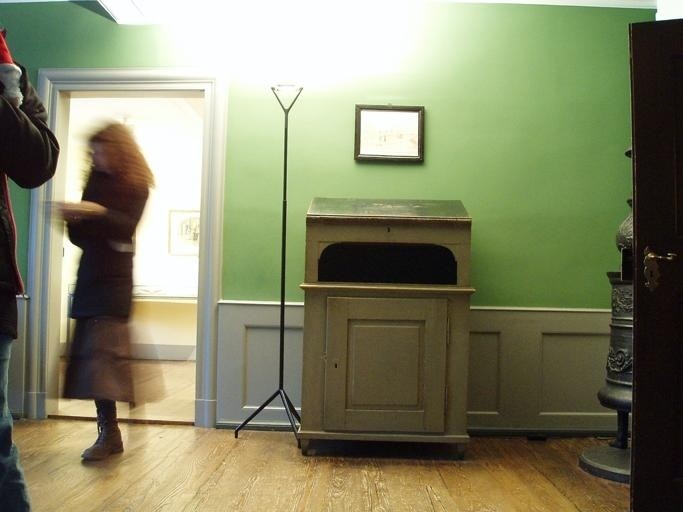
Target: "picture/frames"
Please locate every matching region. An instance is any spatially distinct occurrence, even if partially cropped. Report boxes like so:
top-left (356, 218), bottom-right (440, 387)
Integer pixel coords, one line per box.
top-left (351, 100), bottom-right (426, 169)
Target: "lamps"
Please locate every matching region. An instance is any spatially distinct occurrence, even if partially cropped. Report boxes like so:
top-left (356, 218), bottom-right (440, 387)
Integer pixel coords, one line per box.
top-left (232, 79), bottom-right (306, 448)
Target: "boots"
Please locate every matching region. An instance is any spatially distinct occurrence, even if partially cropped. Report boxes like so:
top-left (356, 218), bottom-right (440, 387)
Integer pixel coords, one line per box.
top-left (82, 409), bottom-right (123, 461)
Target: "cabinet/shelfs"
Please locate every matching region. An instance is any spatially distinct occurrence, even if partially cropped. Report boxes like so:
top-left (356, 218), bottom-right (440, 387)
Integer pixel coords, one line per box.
top-left (293, 196), bottom-right (479, 454)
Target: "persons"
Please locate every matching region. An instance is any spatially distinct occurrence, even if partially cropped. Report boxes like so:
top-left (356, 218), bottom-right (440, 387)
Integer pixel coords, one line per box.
top-left (0, 26), bottom-right (62, 511)
top-left (51, 117), bottom-right (156, 463)
